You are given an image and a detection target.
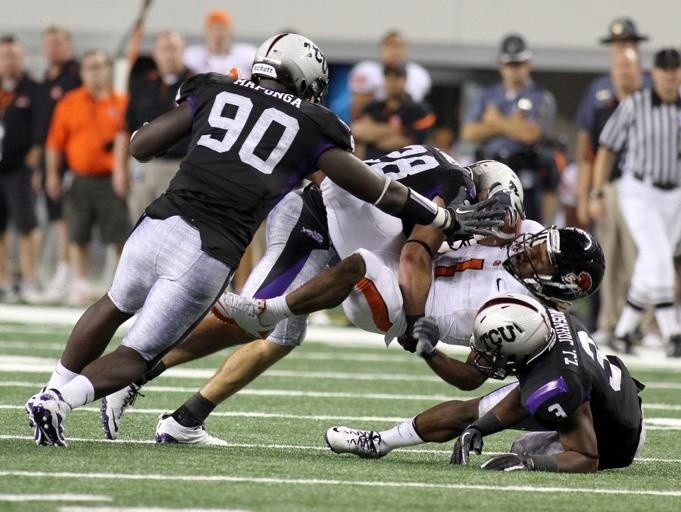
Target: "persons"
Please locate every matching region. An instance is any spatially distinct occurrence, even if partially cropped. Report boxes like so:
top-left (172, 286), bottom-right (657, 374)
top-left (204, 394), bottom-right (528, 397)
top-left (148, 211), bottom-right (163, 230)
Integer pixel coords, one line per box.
top-left (25, 31), bottom-right (645, 475)
top-left (0, 1), bottom-right (680, 358)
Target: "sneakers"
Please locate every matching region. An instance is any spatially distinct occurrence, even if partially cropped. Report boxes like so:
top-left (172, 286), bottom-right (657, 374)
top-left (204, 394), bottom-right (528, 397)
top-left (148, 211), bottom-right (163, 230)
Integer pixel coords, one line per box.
top-left (325, 425), bottom-right (390, 460)
top-left (25, 385), bottom-right (72, 447)
top-left (211, 291), bottom-right (276, 340)
top-left (100, 380), bottom-right (141, 440)
top-left (155, 412), bottom-right (229, 448)
top-left (665, 333), bottom-right (681, 357)
top-left (0, 276), bottom-right (95, 308)
top-left (595, 321), bottom-right (643, 353)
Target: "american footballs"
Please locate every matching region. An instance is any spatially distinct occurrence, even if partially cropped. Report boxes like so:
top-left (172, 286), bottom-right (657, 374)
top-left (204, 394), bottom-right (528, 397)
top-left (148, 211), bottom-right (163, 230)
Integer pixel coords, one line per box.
top-left (477, 208), bottom-right (521, 247)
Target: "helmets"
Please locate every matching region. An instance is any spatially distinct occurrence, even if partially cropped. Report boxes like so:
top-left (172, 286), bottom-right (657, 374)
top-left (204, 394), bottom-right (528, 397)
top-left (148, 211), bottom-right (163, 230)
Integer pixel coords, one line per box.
top-left (469, 293), bottom-right (558, 380)
top-left (507, 224), bottom-right (605, 311)
top-left (500, 36), bottom-right (529, 65)
top-left (467, 159), bottom-right (528, 227)
top-left (252, 32), bottom-right (331, 102)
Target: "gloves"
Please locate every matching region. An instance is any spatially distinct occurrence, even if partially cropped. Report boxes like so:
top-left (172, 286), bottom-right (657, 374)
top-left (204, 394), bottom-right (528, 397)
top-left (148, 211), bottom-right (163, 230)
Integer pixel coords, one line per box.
top-left (397, 312), bottom-right (441, 354)
top-left (450, 424), bottom-right (485, 466)
top-left (444, 186), bottom-right (526, 239)
top-left (480, 452), bottom-right (535, 472)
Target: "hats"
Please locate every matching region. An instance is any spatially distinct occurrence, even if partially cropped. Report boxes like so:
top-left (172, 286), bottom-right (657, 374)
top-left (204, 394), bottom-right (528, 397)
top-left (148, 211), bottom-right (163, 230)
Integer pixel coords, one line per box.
top-left (655, 49), bottom-right (681, 71)
top-left (600, 20), bottom-right (648, 44)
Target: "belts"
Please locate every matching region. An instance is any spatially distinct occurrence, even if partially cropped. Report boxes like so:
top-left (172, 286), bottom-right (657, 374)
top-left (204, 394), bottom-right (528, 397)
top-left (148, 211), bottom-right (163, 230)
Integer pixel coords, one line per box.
top-left (635, 172), bottom-right (677, 190)
